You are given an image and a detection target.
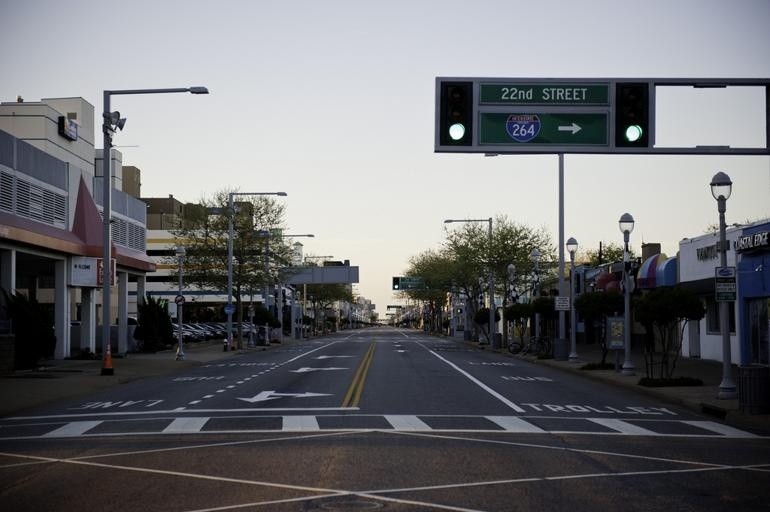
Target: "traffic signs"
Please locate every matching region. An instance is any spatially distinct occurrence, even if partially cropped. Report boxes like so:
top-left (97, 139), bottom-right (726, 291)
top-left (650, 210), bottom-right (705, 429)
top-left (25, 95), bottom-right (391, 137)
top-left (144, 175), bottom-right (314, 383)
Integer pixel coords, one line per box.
top-left (400, 276), bottom-right (426, 290)
top-left (477, 78), bottom-right (615, 152)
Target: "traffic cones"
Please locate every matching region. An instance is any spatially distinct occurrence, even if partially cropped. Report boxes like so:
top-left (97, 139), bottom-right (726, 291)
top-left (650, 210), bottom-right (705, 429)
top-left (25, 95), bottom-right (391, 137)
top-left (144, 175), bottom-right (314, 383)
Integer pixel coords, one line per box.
top-left (101, 343), bottom-right (113, 375)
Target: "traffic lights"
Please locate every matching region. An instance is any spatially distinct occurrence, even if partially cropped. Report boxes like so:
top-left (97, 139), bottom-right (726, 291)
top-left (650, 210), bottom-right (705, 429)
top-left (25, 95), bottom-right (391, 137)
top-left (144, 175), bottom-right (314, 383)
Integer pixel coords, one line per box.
top-left (447, 292), bottom-right (451, 310)
top-left (393, 276), bottom-right (399, 290)
top-left (615, 79), bottom-right (654, 150)
top-left (434, 76), bottom-right (476, 151)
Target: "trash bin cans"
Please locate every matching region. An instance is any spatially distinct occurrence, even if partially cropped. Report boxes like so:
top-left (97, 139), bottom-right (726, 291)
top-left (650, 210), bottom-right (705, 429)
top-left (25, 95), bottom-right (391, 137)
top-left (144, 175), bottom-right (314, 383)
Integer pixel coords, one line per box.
top-left (737, 363), bottom-right (770, 415)
top-left (554, 339), bottom-right (568, 360)
top-left (493, 333), bottom-right (502, 348)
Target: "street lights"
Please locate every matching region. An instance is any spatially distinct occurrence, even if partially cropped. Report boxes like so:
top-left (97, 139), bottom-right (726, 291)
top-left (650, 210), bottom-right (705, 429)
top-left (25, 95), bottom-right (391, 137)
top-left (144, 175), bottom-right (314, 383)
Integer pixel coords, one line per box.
top-left (711, 171), bottom-right (739, 402)
top-left (174, 246), bottom-right (186, 365)
top-left (265, 235), bottom-right (313, 346)
top-left (302, 256), bottom-right (334, 338)
top-left (444, 218), bottom-right (496, 334)
top-left (99, 86), bottom-right (210, 374)
top-left (567, 238), bottom-right (584, 363)
top-left (224, 192), bottom-right (287, 353)
top-left (529, 249), bottom-right (541, 342)
top-left (507, 263), bottom-right (517, 340)
top-left (618, 212), bottom-right (638, 375)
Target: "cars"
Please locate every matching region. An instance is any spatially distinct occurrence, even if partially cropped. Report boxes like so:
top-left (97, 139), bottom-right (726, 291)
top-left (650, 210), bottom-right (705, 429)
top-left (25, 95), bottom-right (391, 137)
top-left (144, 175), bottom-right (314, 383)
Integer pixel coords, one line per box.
top-left (54, 319), bottom-right (82, 329)
top-left (171, 320), bottom-right (261, 344)
